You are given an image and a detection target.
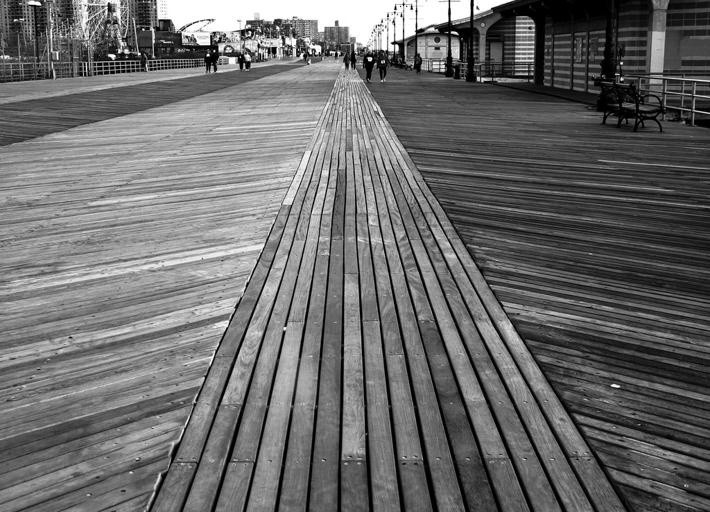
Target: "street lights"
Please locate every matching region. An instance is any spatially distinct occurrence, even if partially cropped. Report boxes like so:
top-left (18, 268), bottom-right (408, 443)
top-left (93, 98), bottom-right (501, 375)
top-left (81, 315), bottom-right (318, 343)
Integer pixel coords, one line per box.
top-left (26, 0), bottom-right (41, 80)
top-left (368, 1), bottom-right (413, 64)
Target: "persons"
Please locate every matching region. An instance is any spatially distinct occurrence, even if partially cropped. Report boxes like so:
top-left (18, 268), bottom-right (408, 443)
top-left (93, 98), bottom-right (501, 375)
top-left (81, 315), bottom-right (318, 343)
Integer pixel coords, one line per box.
top-left (414, 53), bottom-right (422, 79)
top-left (238, 52), bottom-right (251, 72)
top-left (335, 52), bottom-right (338, 62)
top-left (363, 50), bottom-right (390, 83)
top-left (140, 50), bottom-right (148, 72)
top-left (204, 49), bottom-right (220, 74)
top-left (303, 54), bottom-right (308, 62)
top-left (343, 50), bottom-right (357, 72)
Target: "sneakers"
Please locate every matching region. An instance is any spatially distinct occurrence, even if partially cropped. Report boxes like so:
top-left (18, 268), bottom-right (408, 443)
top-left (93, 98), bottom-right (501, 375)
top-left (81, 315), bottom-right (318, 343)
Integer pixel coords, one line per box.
top-left (367, 78), bottom-right (386, 83)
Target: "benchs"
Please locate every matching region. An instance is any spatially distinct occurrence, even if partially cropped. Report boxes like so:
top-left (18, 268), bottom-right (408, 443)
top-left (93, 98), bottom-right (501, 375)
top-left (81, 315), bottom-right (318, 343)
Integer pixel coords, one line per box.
top-left (597, 81), bottom-right (663, 132)
